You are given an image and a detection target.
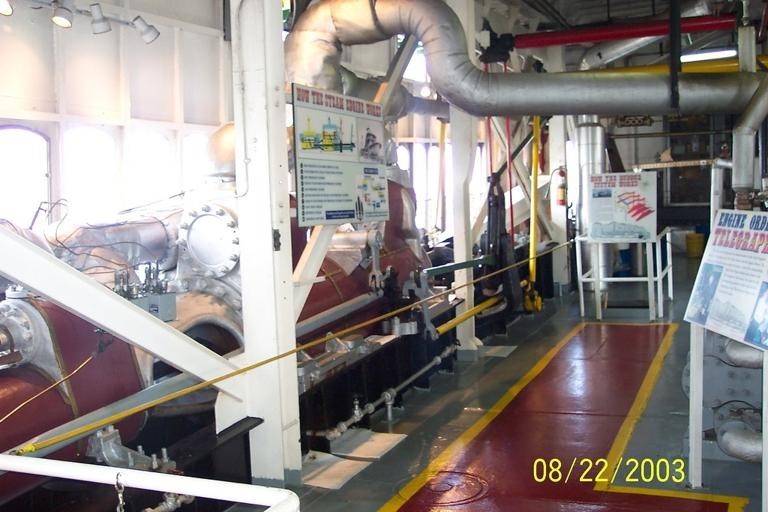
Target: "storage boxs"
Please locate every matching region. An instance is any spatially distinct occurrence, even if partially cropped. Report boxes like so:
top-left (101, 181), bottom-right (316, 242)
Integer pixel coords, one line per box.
top-left (689, 224), bottom-right (709, 235)
top-left (670, 225), bottom-right (696, 255)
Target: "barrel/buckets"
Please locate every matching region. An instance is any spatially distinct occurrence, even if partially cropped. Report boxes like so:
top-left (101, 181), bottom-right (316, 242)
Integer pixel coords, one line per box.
top-left (614, 244), bottom-right (632, 275)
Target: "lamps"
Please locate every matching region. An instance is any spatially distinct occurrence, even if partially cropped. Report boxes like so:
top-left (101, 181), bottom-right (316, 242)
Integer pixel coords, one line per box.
top-left (127, 15), bottom-right (160, 45)
top-left (80, 2), bottom-right (112, 35)
top-left (679, 47), bottom-right (738, 64)
top-left (51, 0), bottom-right (72, 29)
top-left (0, 0), bottom-right (13, 16)
